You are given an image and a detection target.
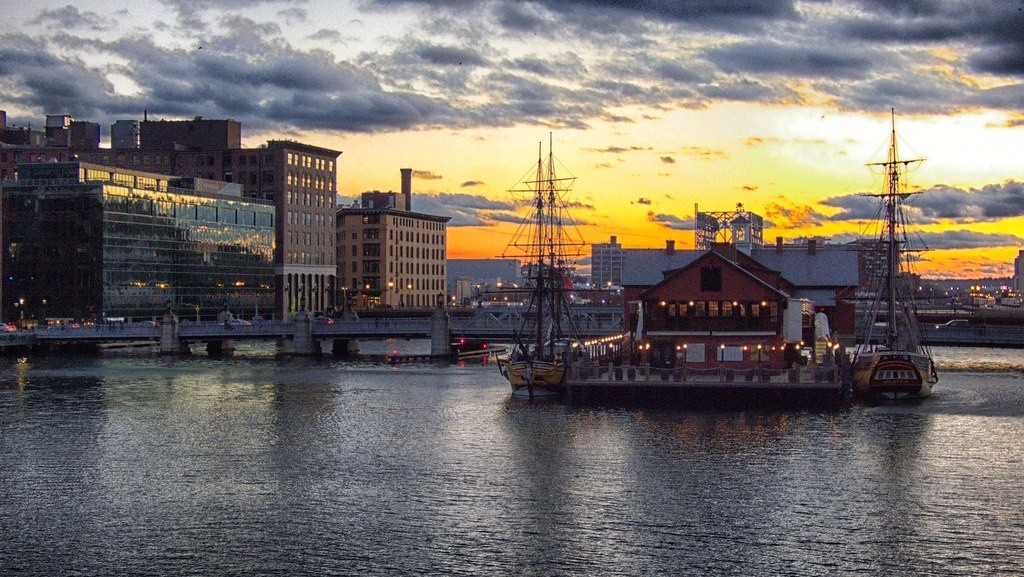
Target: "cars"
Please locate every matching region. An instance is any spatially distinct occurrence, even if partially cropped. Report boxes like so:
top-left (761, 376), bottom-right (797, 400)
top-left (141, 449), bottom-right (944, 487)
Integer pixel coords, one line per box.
top-left (137, 321), bottom-right (156, 327)
top-left (48, 322), bottom-right (80, 330)
top-left (313, 317), bottom-right (334, 323)
top-left (216, 319), bottom-right (251, 325)
top-left (0, 322), bottom-right (16, 333)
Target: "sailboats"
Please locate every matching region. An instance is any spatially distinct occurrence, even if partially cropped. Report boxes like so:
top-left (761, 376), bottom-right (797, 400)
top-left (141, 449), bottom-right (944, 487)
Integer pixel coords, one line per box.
top-left (480, 131), bottom-right (616, 398)
top-left (827, 108), bottom-right (954, 403)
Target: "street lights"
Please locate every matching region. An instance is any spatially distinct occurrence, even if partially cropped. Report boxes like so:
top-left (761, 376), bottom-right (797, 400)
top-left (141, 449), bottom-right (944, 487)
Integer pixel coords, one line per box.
top-left (20, 299), bottom-right (24, 333)
top-left (607, 281), bottom-right (611, 306)
top-left (573, 334), bottom-right (623, 362)
top-left (720, 344), bottom-right (725, 383)
top-left (514, 285), bottom-right (518, 307)
top-left (497, 280), bottom-right (502, 307)
top-left (388, 282), bottom-right (393, 312)
top-left (477, 284), bottom-right (480, 302)
top-left (408, 284), bottom-right (412, 307)
top-left (758, 345), bottom-right (762, 383)
top-left (609, 343), bottom-right (613, 382)
top-left (366, 284), bottom-right (370, 310)
top-left (683, 344), bottom-right (687, 384)
